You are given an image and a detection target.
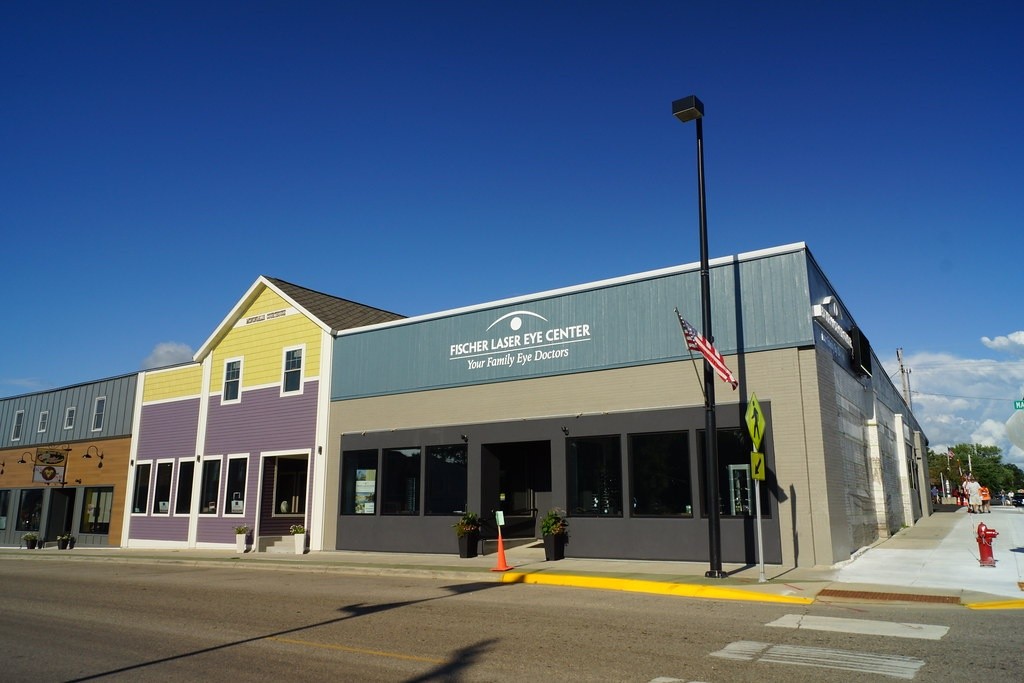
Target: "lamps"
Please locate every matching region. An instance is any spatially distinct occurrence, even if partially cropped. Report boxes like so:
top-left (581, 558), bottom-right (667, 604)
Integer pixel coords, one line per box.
top-left (460, 435), bottom-right (469, 442)
top-left (318, 446), bottom-right (321, 454)
top-left (82, 446), bottom-right (103, 460)
top-left (130, 460), bottom-right (134, 465)
top-left (18, 451), bottom-right (35, 463)
top-left (562, 427), bottom-right (570, 436)
top-left (197, 455), bottom-right (200, 460)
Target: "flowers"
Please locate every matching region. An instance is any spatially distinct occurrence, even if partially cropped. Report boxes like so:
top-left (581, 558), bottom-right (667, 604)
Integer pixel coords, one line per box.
top-left (56, 533), bottom-right (71, 540)
top-left (22, 532), bottom-right (39, 540)
top-left (233, 526), bottom-right (251, 535)
top-left (291, 524), bottom-right (305, 535)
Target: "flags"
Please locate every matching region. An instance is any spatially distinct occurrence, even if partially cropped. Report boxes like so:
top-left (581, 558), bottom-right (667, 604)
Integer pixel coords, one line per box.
top-left (678, 310), bottom-right (738, 391)
top-left (948, 448), bottom-right (955, 457)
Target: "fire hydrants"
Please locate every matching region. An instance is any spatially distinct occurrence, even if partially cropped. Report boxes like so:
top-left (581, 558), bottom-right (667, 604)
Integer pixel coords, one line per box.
top-left (975, 521), bottom-right (999, 567)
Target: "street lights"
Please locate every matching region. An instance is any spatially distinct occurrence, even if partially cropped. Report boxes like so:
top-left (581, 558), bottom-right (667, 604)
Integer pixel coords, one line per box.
top-left (671, 93), bottom-right (729, 580)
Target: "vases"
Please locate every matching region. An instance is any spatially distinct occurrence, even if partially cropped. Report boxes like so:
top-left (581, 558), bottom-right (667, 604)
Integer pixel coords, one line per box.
top-left (56, 539), bottom-right (69, 549)
top-left (295, 534), bottom-right (306, 554)
top-left (27, 539), bottom-right (37, 549)
top-left (236, 533), bottom-right (247, 554)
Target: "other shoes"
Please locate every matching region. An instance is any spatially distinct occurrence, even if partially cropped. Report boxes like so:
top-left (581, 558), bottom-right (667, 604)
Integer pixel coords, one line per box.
top-left (968, 509), bottom-right (990, 514)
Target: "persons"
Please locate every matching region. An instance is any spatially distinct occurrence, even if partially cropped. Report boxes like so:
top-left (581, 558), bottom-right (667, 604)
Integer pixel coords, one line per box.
top-left (1000, 489), bottom-right (1014, 506)
top-left (931, 486), bottom-right (939, 504)
top-left (979, 484), bottom-right (991, 513)
top-left (953, 476), bottom-right (982, 513)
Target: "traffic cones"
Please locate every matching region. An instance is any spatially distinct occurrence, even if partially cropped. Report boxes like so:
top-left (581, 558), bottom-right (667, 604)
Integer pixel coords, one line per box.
top-left (957, 496), bottom-right (961, 506)
top-left (490, 535), bottom-right (514, 572)
top-left (963, 497), bottom-right (968, 506)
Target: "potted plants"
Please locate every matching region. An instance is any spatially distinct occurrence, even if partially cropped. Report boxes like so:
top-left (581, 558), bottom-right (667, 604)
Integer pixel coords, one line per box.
top-left (539, 510), bottom-right (569, 561)
top-left (451, 512), bottom-right (483, 559)
